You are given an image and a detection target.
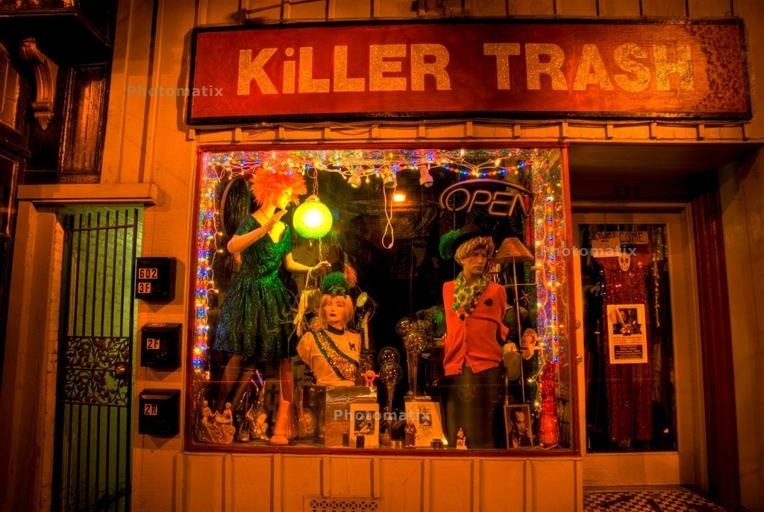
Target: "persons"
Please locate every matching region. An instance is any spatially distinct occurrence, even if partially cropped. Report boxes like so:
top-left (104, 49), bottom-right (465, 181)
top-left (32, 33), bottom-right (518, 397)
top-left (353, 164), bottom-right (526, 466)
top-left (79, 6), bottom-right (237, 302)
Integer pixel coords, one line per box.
top-left (213, 160), bottom-right (541, 448)
top-left (613, 309), bottom-right (630, 334)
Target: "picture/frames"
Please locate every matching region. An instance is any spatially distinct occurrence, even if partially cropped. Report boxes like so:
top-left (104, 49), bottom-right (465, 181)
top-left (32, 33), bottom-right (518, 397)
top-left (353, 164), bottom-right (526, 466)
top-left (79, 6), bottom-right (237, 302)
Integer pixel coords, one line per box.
top-left (502, 402), bottom-right (532, 448)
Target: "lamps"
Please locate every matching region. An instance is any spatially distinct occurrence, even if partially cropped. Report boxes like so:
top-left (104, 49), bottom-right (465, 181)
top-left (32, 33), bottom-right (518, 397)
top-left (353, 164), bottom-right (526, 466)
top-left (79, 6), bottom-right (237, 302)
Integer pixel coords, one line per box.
top-left (290, 148), bottom-right (333, 251)
top-left (343, 149), bottom-right (435, 193)
top-left (490, 233), bottom-right (542, 403)
